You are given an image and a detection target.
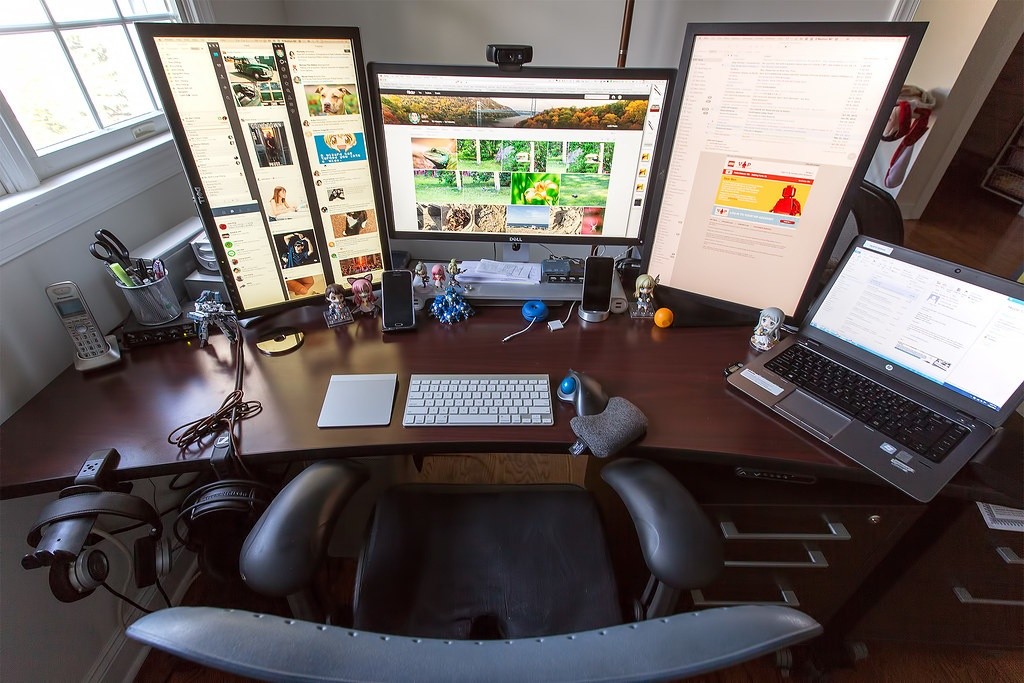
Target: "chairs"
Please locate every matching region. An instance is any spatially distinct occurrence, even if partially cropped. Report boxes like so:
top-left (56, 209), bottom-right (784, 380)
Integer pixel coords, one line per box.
top-left (124, 360), bottom-right (821, 683)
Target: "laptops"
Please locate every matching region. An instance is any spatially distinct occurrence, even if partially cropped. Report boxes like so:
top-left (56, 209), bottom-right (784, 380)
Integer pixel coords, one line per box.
top-left (727, 234), bottom-right (1024, 503)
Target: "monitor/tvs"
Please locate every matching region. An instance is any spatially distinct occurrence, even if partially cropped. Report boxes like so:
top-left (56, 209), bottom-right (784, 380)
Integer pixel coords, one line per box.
top-left (367, 62), bottom-right (678, 301)
top-left (637, 21), bottom-right (931, 328)
top-left (134, 21), bottom-right (391, 329)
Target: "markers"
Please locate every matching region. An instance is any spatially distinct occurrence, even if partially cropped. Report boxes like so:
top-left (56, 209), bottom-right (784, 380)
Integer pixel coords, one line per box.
top-left (112, 264), bottom-right (135, 287)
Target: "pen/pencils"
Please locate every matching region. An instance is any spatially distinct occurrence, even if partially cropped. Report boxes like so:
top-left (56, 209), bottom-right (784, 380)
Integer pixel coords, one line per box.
top-left (127, 258), bottom-right (165, 285)
top-left (104, 263), bottom-right (125, 285)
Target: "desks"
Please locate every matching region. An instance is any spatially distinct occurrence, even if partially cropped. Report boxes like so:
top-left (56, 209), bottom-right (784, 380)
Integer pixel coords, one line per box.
top-left (0, 288), bottom-right (1023, 679)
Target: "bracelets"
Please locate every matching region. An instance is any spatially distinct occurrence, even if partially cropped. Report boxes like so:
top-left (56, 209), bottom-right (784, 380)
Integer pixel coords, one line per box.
top-left (293, 233), bottom-right (296, 236)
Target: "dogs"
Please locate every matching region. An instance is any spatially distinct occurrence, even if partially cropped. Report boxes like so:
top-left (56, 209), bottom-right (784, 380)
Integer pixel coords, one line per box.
top-left (343, 210), bottom-right (368, 236)
top-left (314, 86), bottom-right (352, 115)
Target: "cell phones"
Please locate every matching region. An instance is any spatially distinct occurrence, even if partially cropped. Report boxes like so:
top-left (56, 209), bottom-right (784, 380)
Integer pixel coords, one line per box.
top-left (581, 256), bottom-right (615, 311)
top-left (380, 269), bottom-right (415, 328)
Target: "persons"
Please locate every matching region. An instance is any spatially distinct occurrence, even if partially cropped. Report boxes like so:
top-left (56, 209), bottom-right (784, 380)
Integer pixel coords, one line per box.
top-left (415, 262), bottom-right (430, 288)
top-left (268, 186), bottom-right (297, 222)
top-left (752, 307), bottom-right (787, 350)
top-left (634, 274), bottom-right (656, 313)
top-left (186, 291), bottom-right (237, 348)
top-left (432, 263), bottom-right (446, 288)
top-left (287, 276), bottom-right (314, 296)
top-left (325, 283), bottom-right (349, 318)
top-left (446, 258), bottom-right (466, 288)
top-left (347, 274), bottom-right (379, 318)
top-left (284, 234), bottom-right (315, 268)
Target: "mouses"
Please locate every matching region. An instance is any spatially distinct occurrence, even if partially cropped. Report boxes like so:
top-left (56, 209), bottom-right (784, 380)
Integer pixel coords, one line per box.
top-left (557, 370), bottom-right (610, 417)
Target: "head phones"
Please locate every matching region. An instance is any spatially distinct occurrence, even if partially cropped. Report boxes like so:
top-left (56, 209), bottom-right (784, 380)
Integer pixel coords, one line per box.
top-left (173, 479), bottom-right (277, 583)
top-left (26, 491), bottom-right (171, 603)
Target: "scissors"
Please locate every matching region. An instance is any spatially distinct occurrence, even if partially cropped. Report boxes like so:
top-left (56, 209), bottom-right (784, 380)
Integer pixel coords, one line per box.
top-left (89, 228), bottom-right (135, 270)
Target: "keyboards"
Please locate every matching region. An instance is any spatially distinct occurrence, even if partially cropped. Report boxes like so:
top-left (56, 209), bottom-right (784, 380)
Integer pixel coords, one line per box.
top-left (402, 374), bottom-right (554, 426)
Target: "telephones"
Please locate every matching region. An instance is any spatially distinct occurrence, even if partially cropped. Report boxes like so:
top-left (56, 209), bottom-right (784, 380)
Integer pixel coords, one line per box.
top-left (44, 281), bottom-right (121, 372)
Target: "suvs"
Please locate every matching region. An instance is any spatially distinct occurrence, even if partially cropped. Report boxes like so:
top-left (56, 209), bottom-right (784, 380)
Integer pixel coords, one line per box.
top-left (234, 55), bottom-right (274, 82)
